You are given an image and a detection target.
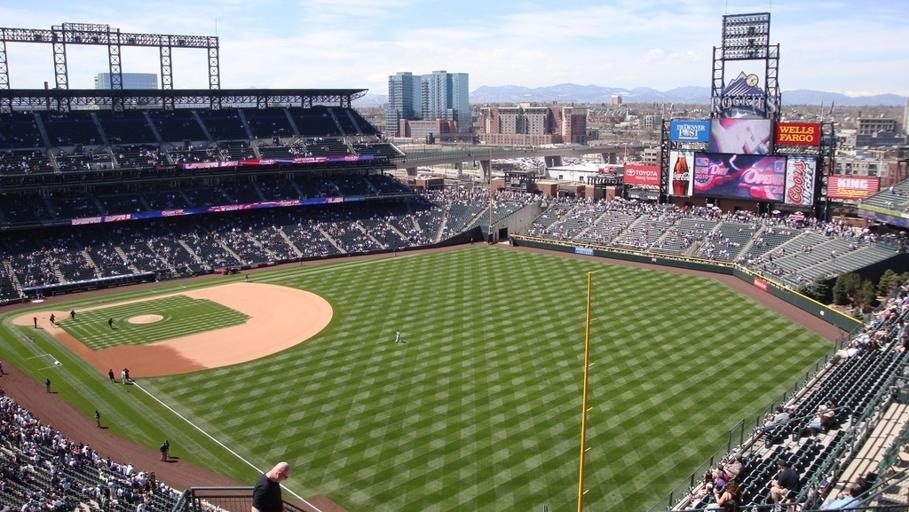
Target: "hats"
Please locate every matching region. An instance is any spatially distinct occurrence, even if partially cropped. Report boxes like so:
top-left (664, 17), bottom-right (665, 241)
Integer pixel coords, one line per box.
top-left (777, 460), bottom-right (786, 465)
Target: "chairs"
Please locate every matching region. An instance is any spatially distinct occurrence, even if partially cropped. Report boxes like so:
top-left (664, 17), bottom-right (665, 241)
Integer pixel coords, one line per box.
top-left (696, 430), bottom-right (850, 511)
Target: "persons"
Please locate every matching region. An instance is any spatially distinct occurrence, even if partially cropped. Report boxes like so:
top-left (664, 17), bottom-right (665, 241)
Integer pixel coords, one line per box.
top-left (108, 317), bottom-right (113, 328)
top-left (51, 314), bottom-right (55, 320)
top-left (0, 388), bottom-right (222, 511)
top-left (109, 368), bottom-right (116, 383)
top-left (807, 404), bottom-right (835, 438)
top-left (688, 452), bottom-right (744, 511)
top-left (395, 330), bottom-right (400, 343)
top-left (827, 398), bottom-right (840, 415)
top-left (124, 368), bottom-right (129, 380)
top-left (121, 368), bottom-right (125, 384)
top-left (821, 476), bottom-right (869, 511)
top-left (34, 317), bottom-right (36, 328)
top-left (768, 460), bottom-right (800, 505)
top-left (830, 285), bottom-right (909, 363)
top-left (44, 377), bottom-right (51, 392)
top-left (70, 309), bottom-right (75, 319)
top-left (50, 318), bottom-right (54, 327)
top-left (768, 406), bottom-right (791, 427)
top-left (249, 461), bottom-right (290, 511)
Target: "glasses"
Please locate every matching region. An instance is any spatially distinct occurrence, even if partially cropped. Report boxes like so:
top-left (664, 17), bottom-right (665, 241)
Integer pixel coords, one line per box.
top-left (282, 473), bottom-right (288, 479)
